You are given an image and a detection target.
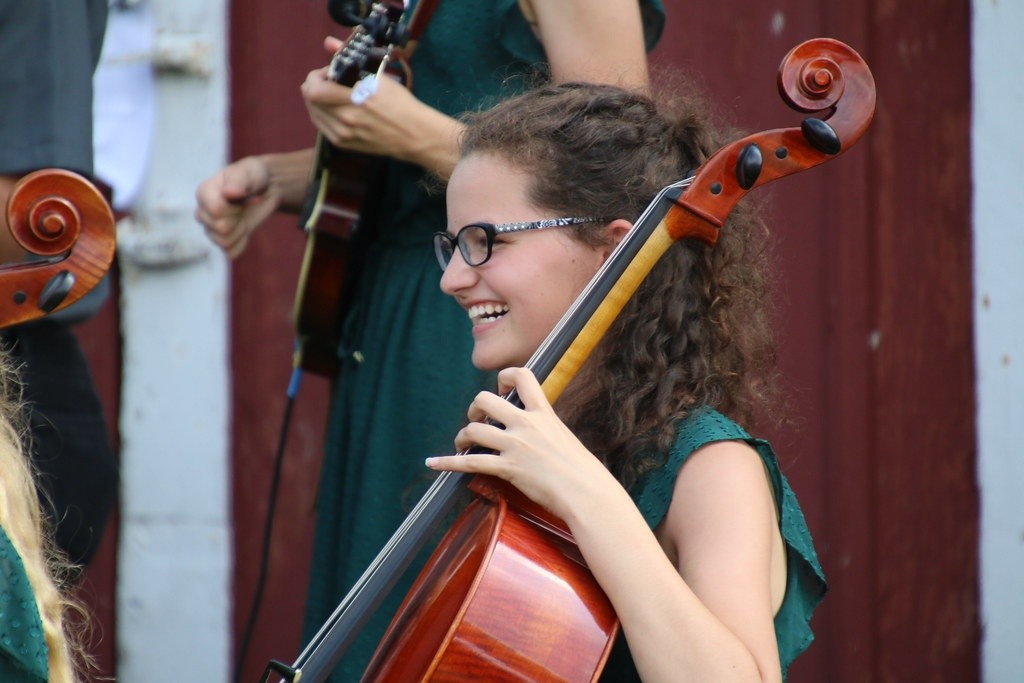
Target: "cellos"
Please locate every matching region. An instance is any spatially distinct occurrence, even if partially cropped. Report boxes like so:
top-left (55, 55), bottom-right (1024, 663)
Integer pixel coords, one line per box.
top-left (0, 162), bottom-right (122, 343)
top-left (255, 29), bottom-right (883, 683)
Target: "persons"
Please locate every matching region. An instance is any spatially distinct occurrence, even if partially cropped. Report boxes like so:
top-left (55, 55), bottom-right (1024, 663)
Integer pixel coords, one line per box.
top-left (196, 0), bottom-right (665, 683)
top-left (425, 83), bottom-right (825, 683)
top-left (0, 0), bottom-right (119, 683)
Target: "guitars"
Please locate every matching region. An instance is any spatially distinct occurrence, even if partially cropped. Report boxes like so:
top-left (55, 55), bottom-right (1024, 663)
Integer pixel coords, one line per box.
top-left (284, 0), bottom-right (438, 386)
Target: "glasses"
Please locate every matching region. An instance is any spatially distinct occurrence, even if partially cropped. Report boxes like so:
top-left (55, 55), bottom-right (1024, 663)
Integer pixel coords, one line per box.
top-left (432, 215), bottom-right (618, 271)
